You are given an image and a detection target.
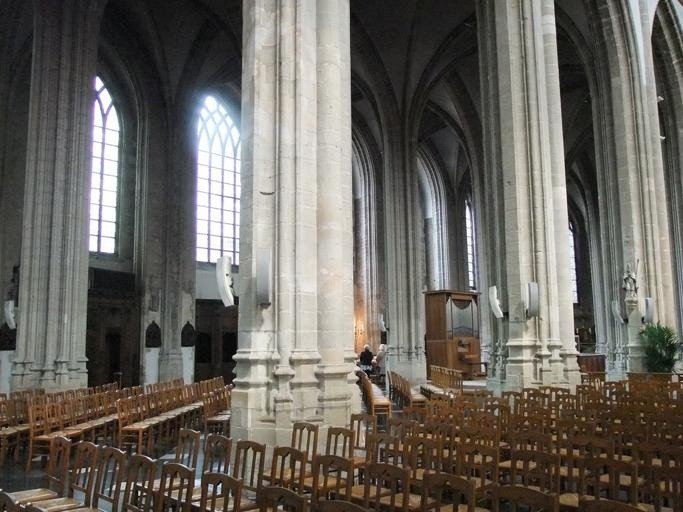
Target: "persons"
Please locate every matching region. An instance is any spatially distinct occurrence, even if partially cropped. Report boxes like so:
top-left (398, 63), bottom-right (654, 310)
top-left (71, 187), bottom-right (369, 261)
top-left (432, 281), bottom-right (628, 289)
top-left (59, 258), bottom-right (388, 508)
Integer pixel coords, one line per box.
top-left (622, 263), bottom-right (636, 298)
top-left (360, 344), bottom-right (373, 378)
top-left (375, 345), bottom-right (386, 384)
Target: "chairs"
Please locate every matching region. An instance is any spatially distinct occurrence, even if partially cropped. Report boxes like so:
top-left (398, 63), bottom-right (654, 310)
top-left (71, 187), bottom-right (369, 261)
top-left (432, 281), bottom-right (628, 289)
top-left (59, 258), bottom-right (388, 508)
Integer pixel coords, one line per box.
top-left (357, 365), bottom-right (465, 425)
top-left (1, 371), bottom-right (681, 512)
top-left (1, 365), bottom-right (234, 477)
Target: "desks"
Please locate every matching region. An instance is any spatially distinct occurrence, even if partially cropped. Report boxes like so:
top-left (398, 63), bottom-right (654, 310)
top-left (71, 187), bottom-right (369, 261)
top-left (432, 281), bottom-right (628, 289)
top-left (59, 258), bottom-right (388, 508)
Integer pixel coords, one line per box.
top-left (578, 352), bottom-right (606, 381)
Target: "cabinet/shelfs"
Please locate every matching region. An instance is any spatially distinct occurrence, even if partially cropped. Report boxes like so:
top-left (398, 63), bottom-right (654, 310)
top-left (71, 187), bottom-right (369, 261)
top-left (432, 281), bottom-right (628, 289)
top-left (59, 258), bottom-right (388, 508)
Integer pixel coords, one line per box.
top-left (422, 290), bottom-right (482, 380)
